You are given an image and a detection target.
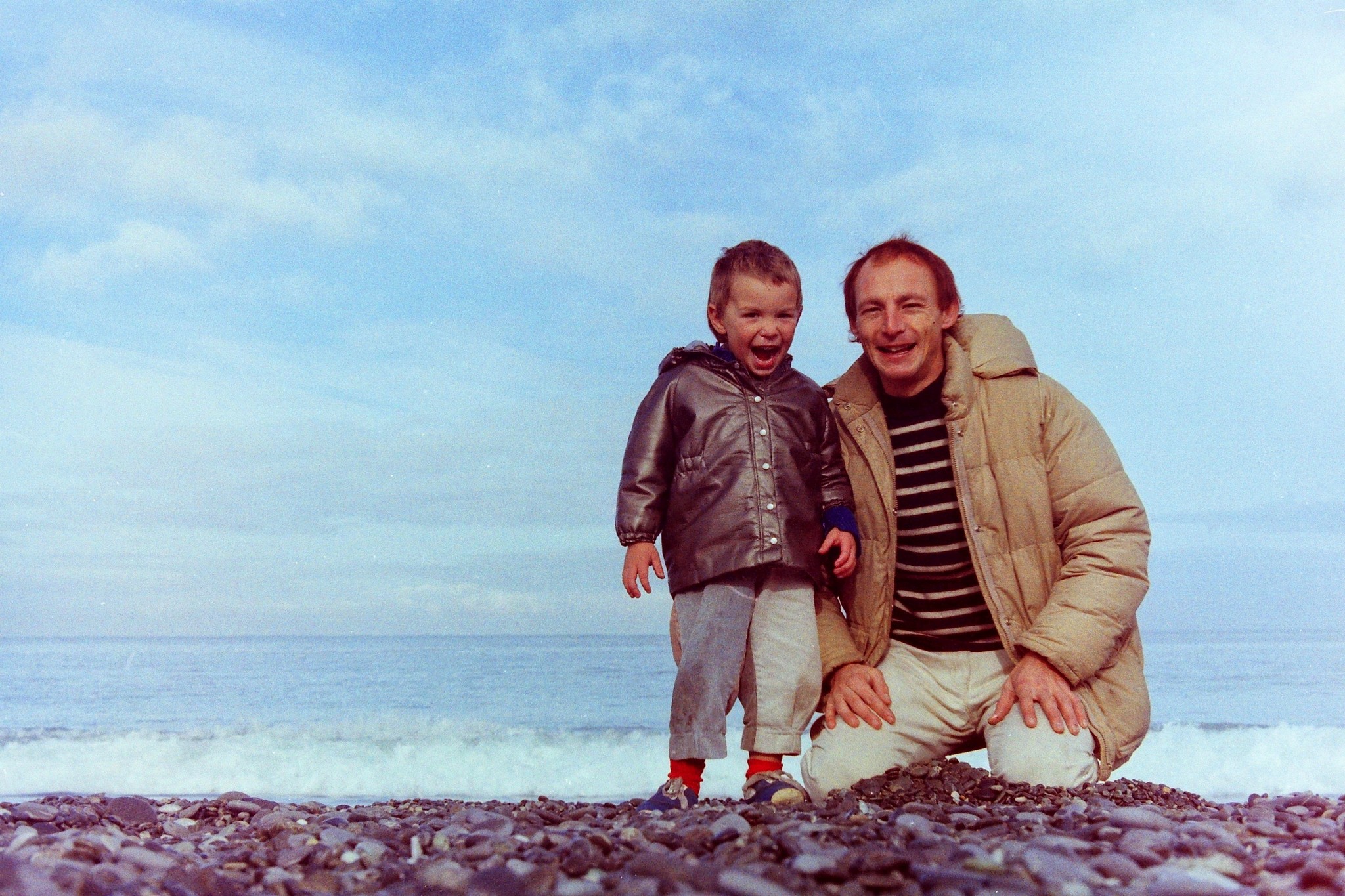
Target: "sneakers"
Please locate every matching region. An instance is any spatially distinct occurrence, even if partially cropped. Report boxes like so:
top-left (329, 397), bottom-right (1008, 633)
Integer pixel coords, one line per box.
top-left (635, 781), bottom-right (698, 813)
top-left (744, 769), bottom-right (809, 803)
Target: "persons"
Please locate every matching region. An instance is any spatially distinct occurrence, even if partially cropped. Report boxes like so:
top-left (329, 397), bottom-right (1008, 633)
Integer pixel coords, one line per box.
top-left (799, 236), bottom-right (1151, 809)
top-left (614, 240), bottom-right (860, 814)
